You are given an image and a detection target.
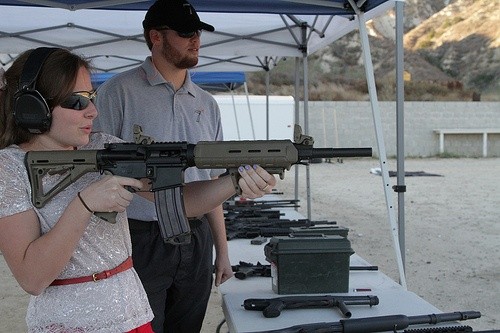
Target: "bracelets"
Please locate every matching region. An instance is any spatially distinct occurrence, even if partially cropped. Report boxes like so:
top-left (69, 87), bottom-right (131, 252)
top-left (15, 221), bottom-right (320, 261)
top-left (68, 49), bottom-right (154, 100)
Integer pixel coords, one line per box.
top-left (78, 192), bottom-right (93, 213)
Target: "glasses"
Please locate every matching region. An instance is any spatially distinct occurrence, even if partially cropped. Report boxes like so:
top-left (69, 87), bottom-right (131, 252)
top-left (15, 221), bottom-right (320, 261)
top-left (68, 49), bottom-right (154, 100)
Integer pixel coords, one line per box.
top-left (58, 88), bottom-right (99, 110)
top-left (161, 27), bottom-right (203, 38)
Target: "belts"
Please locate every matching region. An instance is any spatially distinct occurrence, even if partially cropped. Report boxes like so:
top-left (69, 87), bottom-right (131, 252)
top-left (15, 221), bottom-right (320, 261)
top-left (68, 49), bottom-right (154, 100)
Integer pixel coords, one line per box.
top-left (49, 256), bottom-right (133, 285)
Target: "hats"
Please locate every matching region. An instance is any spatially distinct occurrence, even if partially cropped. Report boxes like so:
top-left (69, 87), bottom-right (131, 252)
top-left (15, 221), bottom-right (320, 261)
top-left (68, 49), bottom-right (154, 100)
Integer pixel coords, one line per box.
top-left (143, 0), bottom-right (214, 32)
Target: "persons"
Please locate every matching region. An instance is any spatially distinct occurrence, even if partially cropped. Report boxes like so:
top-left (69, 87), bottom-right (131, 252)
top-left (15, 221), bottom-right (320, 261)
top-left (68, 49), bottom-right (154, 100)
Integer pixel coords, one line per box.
top-left (0, 47), bottom-right (277, 332)
top-left (89, 0), bottom-right (235, 333)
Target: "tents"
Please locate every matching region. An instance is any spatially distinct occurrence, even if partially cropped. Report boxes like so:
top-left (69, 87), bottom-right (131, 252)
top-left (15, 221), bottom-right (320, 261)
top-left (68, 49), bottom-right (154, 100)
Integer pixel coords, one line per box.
top-left (91, 72), bottom-right (257, 141)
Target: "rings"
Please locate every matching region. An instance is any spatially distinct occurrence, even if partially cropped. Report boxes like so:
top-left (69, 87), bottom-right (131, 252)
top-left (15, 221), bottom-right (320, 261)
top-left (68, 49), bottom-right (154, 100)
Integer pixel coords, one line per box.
top-left (262, 184), bottom-right (269, 191)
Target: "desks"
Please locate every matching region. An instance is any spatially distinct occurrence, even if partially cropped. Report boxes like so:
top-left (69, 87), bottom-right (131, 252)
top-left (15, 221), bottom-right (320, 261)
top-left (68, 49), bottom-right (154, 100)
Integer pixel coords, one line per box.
top-left (433, 128), bottom-right (500, 158)
top-left (212, 195), bottom-right (463, 333)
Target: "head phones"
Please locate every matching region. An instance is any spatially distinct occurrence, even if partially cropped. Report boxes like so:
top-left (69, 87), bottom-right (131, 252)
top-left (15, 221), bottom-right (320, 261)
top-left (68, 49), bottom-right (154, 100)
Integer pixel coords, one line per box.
top-left (13, 47), bottom-right (60, 134)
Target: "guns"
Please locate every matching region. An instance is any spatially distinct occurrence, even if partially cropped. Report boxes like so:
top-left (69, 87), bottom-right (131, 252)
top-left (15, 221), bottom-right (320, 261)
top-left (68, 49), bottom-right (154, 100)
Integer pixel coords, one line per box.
top-left (221, 189), bottom-right (337, 239)
top-left (236, 309), bottom-right (483, 333)
top-left (23, 124), bottom-right (373, 245)
top-left (231, 261), bottom-right (378, 280)
top-left (243, 294), bottom-right (380, 318)
top-left (401, 326), bottom-right (500, 333)
